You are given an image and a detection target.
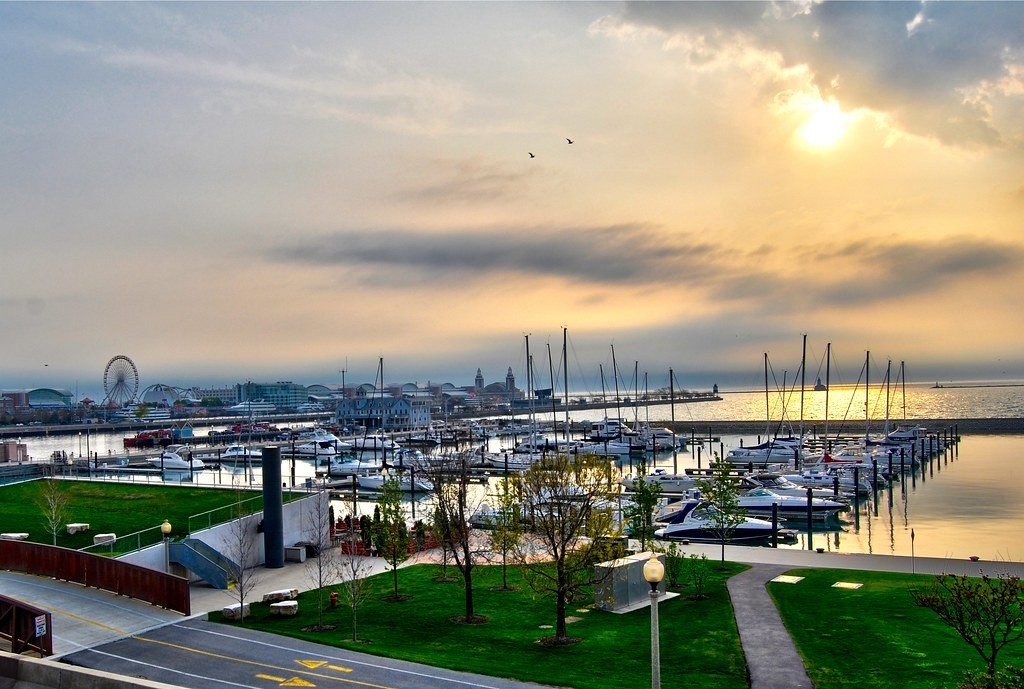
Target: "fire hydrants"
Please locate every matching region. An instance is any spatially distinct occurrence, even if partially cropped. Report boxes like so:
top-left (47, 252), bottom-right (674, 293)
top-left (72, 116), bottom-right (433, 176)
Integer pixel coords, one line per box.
top-left (330, 592), bottom-right (339, 608)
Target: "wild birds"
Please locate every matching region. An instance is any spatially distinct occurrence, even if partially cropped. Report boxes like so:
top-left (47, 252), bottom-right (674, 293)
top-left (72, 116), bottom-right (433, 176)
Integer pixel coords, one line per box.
top-left (528, 153), bottom-right (535, 158)
top-left (566, 138), bottom-right (574, 144)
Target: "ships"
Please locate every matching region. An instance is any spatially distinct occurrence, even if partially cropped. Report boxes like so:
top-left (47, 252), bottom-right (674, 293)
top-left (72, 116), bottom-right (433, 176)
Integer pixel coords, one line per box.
top-left (229, 399), bottom-right (277, 413)
top-left (110, 398), bottom-right (171, 421)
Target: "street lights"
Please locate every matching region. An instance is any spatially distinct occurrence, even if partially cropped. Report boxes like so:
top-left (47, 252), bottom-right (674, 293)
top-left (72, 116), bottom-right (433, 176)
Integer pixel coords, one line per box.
top-left (161, 518), bottom-right (172, 572)
top-left (642, 552), bottom-right (664, 689)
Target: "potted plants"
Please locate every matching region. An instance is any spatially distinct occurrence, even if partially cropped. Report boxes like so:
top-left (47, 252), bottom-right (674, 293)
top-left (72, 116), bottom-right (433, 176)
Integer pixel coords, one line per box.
top-left (329, 504), bottom-right (470, 557)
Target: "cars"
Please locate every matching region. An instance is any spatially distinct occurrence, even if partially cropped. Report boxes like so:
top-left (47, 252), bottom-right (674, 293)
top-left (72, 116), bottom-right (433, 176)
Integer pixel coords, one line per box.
top-left (208, 427), bottom-right (266, 437)
top-left (269, 427), bottom-right (279, 432)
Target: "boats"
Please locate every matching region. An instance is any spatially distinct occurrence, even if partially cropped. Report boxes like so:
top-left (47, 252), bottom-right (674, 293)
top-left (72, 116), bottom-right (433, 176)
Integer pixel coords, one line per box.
top-left (295, 403), bottom-right (326, 412)
top-left (145, 444), bottom-right (205, 471)
top-left (201, 327), bottom-right (948, 514)
top-left (356, 473), bottom-right (435, 493)
top-left (653, 498), bottom-right (788, 542)
